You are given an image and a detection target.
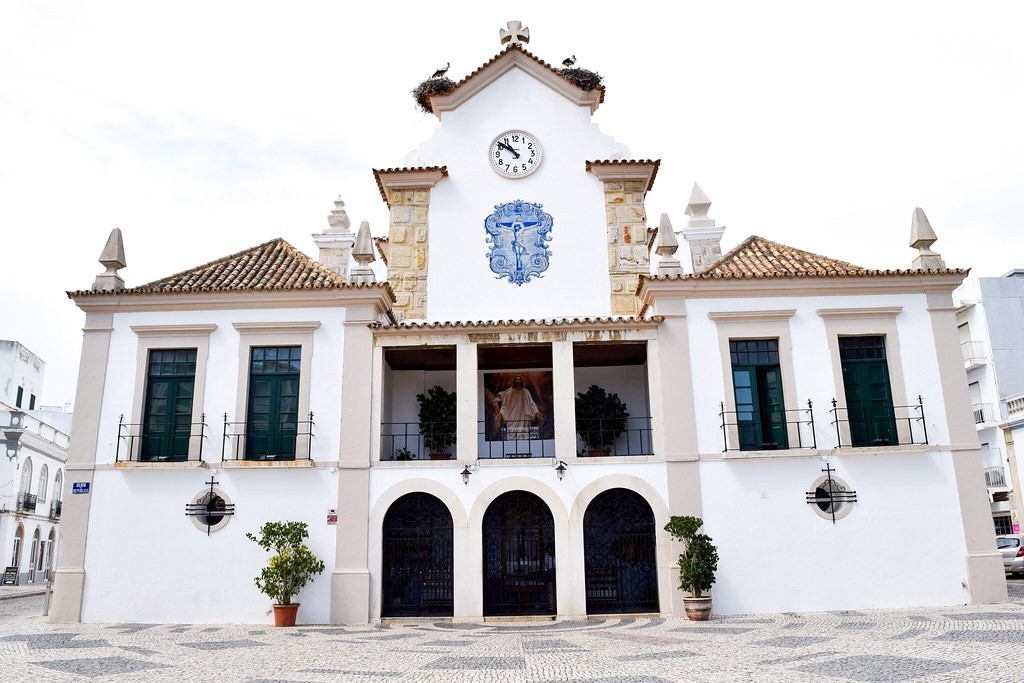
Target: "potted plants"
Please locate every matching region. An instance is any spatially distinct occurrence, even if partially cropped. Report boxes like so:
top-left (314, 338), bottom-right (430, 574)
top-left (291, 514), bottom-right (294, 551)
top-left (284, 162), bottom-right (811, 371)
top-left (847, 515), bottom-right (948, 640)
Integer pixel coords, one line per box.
top-left (245, 520), bottom-right (325, 627)
top-left (416, 385), bottom-right (457, 460)
top-left (575, 384), bottom-right (630, 456)
top-left (665, 515), bottom-right (719, 620)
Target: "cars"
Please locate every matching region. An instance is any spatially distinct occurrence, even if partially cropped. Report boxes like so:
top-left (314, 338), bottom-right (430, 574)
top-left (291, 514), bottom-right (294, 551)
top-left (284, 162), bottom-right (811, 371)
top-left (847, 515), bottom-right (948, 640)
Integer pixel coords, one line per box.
top-left (996, 533), bottom-right (1024, 576)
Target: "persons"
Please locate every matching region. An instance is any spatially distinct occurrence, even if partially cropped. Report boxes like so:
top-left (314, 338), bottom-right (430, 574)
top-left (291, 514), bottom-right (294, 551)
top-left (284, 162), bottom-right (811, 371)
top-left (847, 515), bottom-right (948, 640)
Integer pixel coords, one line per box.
top-left (544, 542), bottom-right (555, 611)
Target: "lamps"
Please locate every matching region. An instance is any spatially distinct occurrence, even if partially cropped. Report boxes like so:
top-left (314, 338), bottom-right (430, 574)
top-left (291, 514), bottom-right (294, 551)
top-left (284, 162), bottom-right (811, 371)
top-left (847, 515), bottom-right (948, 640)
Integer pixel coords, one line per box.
top-left (556, 461), bottom-right (568, 480)
top-left (460, 465), bottom-right (472, 484)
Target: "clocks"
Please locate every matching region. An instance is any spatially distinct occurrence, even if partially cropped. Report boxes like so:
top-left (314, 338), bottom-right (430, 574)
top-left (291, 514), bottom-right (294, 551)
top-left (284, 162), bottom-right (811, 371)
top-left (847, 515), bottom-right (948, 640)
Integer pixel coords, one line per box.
top-left (489, 130), bottom-right (544, 180)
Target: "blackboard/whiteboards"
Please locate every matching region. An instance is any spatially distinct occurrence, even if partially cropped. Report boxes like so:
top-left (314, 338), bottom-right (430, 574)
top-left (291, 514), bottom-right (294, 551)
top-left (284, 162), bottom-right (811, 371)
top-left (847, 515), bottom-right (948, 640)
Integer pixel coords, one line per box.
top-left (2, 567), bottom-right (19, 586)
